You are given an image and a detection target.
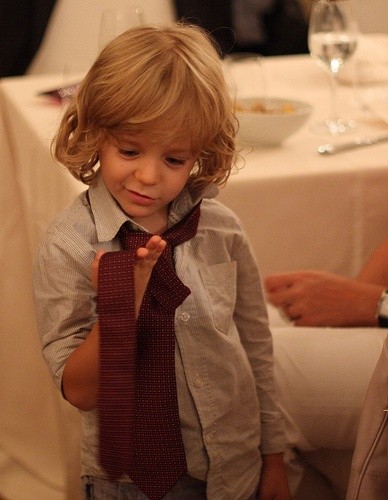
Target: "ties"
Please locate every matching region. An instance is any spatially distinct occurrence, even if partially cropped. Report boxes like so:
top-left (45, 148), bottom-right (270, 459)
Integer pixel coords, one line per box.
top-left (85, 187), bottom-right (203, 500)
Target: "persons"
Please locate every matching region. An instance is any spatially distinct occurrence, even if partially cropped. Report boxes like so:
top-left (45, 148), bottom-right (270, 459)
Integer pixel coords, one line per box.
top-left (245, 228), bottom-right (387, 499)
top-left (25, 18), bottom-right (300, 499)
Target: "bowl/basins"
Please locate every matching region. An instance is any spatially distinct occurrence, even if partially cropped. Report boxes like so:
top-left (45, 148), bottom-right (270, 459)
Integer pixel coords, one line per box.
top-left (231, 95), bottom-right (314, 150)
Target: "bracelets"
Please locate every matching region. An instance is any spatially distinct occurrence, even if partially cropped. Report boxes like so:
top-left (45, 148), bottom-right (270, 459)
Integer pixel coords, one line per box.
top-left (375, 286), bottom-right (387, 330)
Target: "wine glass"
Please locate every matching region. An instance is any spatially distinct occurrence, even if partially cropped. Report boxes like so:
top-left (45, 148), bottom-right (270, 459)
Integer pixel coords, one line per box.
top-left (307, 0), bottom-right (359, 135)
